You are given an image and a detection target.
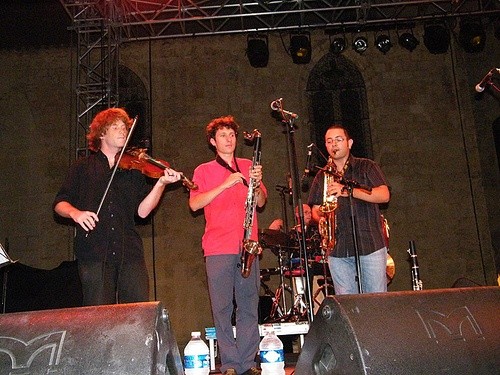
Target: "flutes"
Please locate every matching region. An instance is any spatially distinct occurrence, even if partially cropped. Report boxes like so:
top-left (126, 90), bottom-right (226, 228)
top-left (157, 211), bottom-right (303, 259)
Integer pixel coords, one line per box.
top-left (407, 240), bottom-right (423, 291)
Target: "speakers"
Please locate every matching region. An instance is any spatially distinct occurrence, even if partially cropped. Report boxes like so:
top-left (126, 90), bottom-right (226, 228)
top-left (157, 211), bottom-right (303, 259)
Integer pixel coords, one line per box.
top-left (294, 285), bottom-right (500, 375)
top-left (0, 300), bottom-right (184, 375)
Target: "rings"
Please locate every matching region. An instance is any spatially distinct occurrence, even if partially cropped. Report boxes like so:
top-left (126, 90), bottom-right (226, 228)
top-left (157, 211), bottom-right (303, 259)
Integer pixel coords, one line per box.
top-left (169, 174), bottom-right (174, 177)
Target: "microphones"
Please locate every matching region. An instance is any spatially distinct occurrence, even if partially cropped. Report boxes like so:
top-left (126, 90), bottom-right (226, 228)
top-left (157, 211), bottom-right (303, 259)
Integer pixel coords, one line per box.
top-left (475, 67), bottom-right (498, 93)
top-left (276, 108), bottom-right (298, 119)
top-left (305, 146), bottom-right (314, 175)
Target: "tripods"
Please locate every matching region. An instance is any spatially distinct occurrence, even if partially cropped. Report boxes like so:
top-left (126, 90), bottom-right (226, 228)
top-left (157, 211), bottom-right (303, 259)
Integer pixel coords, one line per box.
top-left (268, 243), bottom-right (310, 323)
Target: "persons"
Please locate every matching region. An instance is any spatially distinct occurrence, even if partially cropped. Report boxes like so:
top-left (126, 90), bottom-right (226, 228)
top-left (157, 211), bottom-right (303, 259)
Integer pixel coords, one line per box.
top-left (189, 118), bottom-right (268, 375)
top-left (293, 204), bottom-right (336, 320)
top-left (307, 126), bottom-right (392, 295)
top-left (52, 108), bottom-right (184, 306)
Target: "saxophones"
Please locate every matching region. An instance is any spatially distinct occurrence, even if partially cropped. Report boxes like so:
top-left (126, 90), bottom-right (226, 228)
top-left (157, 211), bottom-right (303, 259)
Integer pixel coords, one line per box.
top-left (315, 153), bottom-right (339, 255)
top-left (237, 128), bottom-right (263, 278)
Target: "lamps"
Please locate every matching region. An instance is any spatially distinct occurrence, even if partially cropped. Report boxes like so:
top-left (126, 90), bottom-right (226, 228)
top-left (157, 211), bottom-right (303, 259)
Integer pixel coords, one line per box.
top-left (456, 13), bottom-right (486, 54)
top-left (352, 25), bottom-right (368, 55)
top-left (289, 28), bottom-right (312, 64)
top-left (396, 22), bottom-right (419, 52)
top-left (374, 23), bottom-right (393, 55)
top-left (245, 33), bottom-right (269, 68)
top-left (421, 17), bottom-right (452, 54)
top-left (328, 26), bottom-right (349, 56)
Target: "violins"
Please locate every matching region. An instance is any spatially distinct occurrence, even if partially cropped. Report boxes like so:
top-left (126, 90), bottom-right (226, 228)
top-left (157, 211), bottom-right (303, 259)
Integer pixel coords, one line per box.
top-left (115, 149), bottom-right (200, 190)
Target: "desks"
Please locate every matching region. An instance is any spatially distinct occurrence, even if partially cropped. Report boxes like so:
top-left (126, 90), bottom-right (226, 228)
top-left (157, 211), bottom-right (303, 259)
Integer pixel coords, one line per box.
top-left (204, 321), bottom-right (310, 370)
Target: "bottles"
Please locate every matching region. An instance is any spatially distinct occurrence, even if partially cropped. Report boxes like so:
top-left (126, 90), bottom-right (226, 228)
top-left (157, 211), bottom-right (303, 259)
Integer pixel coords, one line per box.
top-left (183, 330), bottom-right (211, 375)
top-left (259, 328), bottom-right (287, 374)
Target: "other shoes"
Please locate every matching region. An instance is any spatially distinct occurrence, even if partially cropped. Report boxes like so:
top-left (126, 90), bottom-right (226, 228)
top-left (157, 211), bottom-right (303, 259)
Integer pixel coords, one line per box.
top-left (224, 368), bottom-right (238, 375)
top-left (242, 368), bottom-right (260, 375)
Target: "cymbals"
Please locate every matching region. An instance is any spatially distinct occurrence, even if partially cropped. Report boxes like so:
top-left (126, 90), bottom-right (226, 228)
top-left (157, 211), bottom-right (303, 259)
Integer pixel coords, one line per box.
top-left (387, 254), bottom-right (395, 284)
top-left (258, 227), bottom-right (300, 248)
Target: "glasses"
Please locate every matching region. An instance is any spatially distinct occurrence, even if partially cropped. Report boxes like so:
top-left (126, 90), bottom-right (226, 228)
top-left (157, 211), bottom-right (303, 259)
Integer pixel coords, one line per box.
top-left (111, 124), bottom-right (129, 131)
top-left (326, 137), bottom-right (349, 144)
